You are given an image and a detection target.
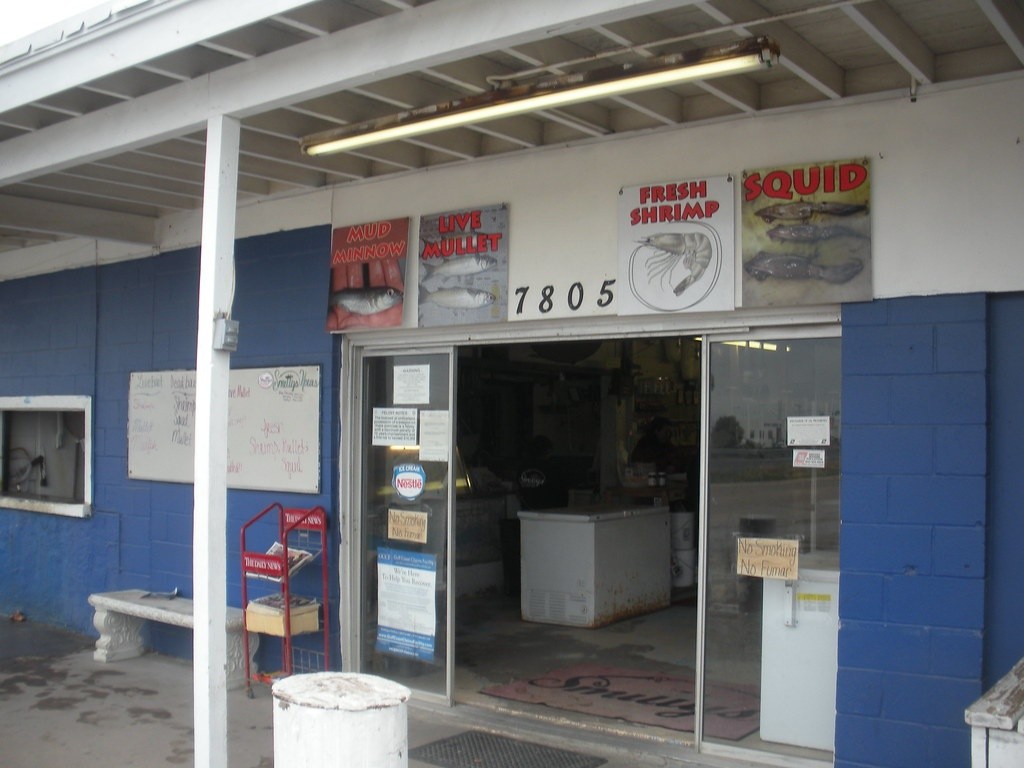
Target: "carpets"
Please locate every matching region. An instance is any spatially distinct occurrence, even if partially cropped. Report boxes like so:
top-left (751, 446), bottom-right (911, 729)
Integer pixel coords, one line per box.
top-left (409, 729), bottom-right (608, 768)
top-left (482, 660), bottom-right (760, 741)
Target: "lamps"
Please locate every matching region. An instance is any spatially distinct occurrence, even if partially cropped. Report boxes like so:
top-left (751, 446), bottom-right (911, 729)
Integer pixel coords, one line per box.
top-left (297, 33), bottom-right (782, 156)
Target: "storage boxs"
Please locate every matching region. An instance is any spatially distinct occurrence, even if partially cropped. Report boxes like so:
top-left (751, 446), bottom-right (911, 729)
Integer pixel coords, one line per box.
top-left (245, 590), bottom-right (322, 635)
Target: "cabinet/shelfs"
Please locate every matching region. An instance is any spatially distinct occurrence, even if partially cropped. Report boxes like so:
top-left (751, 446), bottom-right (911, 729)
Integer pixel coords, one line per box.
top-left (239, 502), bottom-right (330, 689)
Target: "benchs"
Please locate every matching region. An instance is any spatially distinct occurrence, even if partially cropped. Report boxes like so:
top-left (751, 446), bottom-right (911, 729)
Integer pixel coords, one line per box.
top-left (89, 589), bottom-right (261, 689)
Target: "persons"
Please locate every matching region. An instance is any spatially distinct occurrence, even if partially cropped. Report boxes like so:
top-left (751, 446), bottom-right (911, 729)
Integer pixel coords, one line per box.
top-left (629, 416), bottom-right (675, 465)
top-left (515, 435), bottom-right (581, 512)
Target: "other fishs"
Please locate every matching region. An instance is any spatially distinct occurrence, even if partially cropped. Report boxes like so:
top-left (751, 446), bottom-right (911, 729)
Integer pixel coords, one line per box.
top-left (326, 286), bottom-right (401, 317)
top-left (416, 235), bottom-right (502, 310)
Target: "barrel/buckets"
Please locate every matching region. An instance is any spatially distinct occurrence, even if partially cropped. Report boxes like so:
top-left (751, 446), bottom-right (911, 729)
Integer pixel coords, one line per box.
top-left (273, 672), bottom-right (411, 768)
top-left (671, 549), bottom-right (696, 587)
top-left (670, 513), bottom-right (694, 548)
